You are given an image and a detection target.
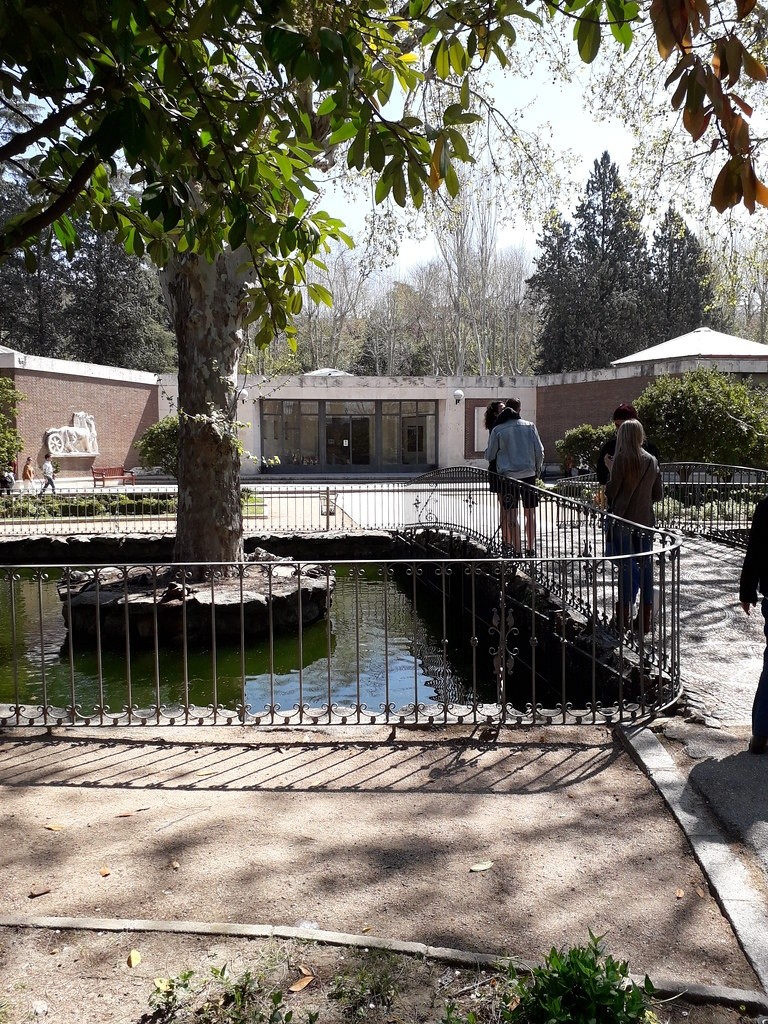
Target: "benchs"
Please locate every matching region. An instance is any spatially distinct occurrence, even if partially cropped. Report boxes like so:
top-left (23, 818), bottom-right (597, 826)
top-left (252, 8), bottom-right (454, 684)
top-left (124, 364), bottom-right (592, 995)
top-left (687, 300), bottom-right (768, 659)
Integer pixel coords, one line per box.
top-left (90, 466), bottom-right (136, 487)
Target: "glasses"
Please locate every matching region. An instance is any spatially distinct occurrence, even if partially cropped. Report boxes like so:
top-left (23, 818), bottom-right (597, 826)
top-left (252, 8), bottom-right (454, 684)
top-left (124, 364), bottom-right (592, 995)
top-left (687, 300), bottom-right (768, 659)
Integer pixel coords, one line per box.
top-left (615, 424), bottom-right (620, 429)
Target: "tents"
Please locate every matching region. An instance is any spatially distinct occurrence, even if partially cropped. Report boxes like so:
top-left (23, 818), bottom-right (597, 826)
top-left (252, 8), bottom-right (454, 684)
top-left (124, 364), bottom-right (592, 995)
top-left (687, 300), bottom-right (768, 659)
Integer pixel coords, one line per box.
top-left (609, 327), bottom-right (768, 367)
top-left (304, 368), bottom-right (354, 376)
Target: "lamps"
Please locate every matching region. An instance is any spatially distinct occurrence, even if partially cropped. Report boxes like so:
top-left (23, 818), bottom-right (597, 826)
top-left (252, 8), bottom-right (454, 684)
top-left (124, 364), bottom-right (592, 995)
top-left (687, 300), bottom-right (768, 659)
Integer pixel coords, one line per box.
top-left (238, 389), bottom-right (248, 405)
top-left (454, 389), bottom-right (464, 405)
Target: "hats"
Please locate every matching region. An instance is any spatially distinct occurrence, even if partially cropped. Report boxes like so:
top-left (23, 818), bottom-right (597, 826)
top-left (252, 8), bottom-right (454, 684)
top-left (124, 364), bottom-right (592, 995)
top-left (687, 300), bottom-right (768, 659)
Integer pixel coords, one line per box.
top-left (613, 404), bottom-right (637, 421)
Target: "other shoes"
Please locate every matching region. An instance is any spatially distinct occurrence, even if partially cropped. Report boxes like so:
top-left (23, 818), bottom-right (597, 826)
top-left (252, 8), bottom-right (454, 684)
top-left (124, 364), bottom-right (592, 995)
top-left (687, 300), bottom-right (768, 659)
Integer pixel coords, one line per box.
top-left (525, 549), bottom-right (538, 559)
top-left (749, 735), bottom-right (768, 755)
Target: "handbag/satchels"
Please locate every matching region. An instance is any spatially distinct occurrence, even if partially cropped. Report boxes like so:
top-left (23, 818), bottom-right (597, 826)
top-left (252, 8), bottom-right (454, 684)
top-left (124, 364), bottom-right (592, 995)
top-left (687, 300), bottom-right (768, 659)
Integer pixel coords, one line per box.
top-left (605, 511), bottom-right (618, 544)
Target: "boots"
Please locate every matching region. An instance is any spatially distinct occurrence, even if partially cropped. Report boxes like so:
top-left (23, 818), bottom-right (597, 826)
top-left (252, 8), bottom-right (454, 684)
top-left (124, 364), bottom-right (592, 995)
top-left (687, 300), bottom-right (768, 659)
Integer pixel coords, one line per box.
top-left (609, 602), bottom-right (633, 634)
top-left (629, 602), bottom-right (653, 634)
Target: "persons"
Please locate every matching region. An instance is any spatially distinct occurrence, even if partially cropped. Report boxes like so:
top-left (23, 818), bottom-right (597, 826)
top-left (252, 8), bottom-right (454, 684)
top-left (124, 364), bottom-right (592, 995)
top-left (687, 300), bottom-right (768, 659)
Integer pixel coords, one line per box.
top-left (22, 457), bottom-right (36, 496)
top-left (739, 495), bottom-right (768, 753)
top-left (604, 419), bottom-right (663, 634)
top-left (485, 397), bottom-right (545, 558)
top-left (8, 458), bottom-right (16, 479)
top-left (596, 403), bottom-right (638, 619)
top-left (42, 454), bottom-right (56, 494)
